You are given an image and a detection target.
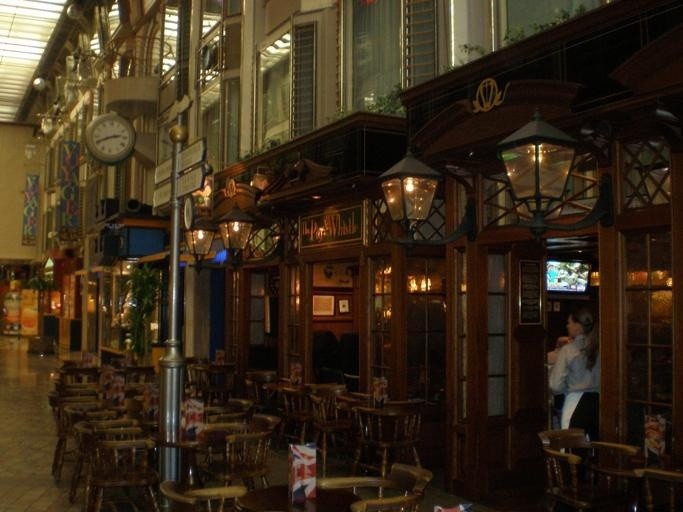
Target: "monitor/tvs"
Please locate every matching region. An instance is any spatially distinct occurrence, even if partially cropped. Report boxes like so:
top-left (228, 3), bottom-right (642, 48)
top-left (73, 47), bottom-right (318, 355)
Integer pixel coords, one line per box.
top-left (544, 259), bottom-right (591, 299)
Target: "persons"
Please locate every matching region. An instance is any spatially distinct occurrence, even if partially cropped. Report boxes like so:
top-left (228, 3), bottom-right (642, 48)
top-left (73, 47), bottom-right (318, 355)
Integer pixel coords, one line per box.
top-left (548, 311), bottom-right (598, 458)
top-left (547, 262), bottom-right (587, 291)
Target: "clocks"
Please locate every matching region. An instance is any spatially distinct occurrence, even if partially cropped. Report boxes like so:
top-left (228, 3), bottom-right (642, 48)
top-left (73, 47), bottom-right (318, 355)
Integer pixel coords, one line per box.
top-left (84, 113), bottom-right (135, 163)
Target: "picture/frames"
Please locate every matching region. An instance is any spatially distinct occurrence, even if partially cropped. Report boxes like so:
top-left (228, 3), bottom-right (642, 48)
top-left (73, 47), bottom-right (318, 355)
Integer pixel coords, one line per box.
top-left (313, 261), bottom-right (356, 292)
top-left (312, 294), bottom-right (335, 317)
top-left (338, 298), bottom-right (351, 315)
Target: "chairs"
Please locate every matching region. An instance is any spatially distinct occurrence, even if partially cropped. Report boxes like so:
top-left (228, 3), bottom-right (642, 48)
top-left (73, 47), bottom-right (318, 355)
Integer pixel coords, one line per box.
top-left (46, 358), bottom-right (437, 512)
top-left (599, 458), bottom-right (681, 511)
top-left (312, 329), bottom-right (359, 392)
top-left (538, 428), bottom-right (638, 510)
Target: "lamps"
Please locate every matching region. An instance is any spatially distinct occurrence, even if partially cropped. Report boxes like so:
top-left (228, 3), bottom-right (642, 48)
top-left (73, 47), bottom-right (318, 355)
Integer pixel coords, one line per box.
top-left (183, 215), bottom-right (226, 275)
top-left (374, 146), bottom-right (475, 246)
top-left (494, 107), bottom-right (614, 242)
top-left (215, 202), bottom-right (280, 271)
